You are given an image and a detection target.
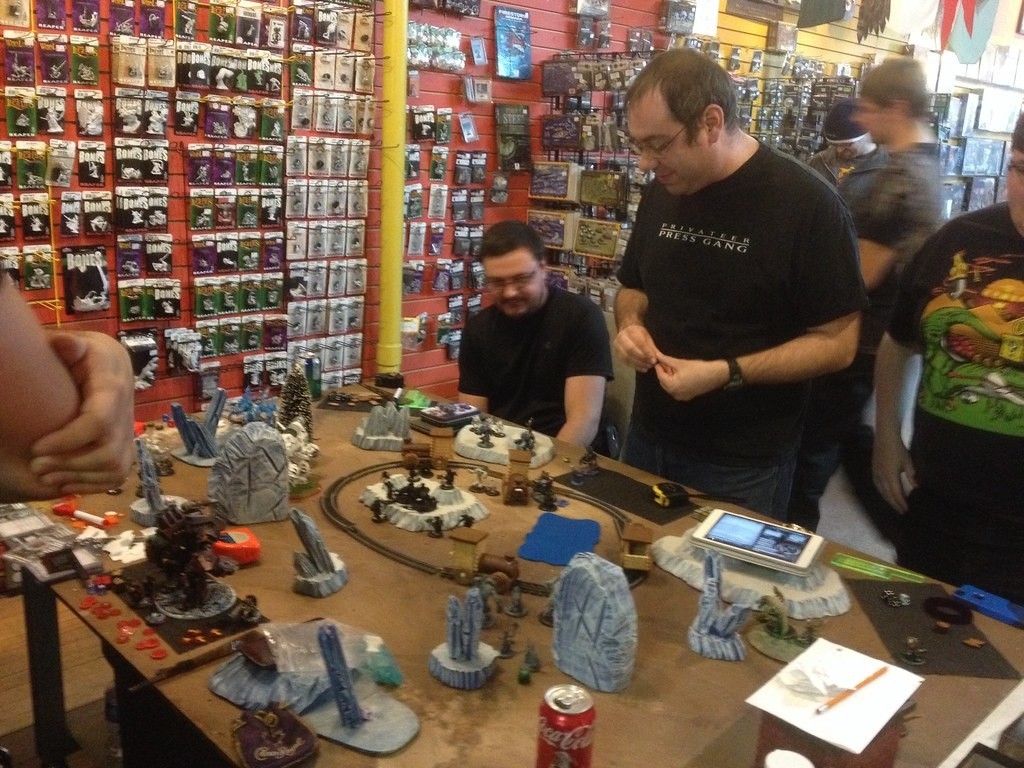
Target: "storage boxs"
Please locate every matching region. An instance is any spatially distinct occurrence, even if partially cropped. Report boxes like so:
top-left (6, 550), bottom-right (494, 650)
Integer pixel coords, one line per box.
top-left (541, 59), bottom-right (593, 96)
top-left (544, 265), bottom-right (578, 295)
top-left (527, 162), bottom-right (626, 208)
top-left (658, 0), bottom-right (696, 35)
top-left (527, 209), bottom-right (623, 261)
top-left (569, 0), bottom-right (609, 21)
top-left (420, 404), bottom-right (481, 428)
top-left (904, 41), bottom-right (1024, 219)
top-left (541, 114), bottom-right (585, 152)
top-left (766, 22), bottom-right (798, 51)
top-left (439, 0), bottom-right (480, 16)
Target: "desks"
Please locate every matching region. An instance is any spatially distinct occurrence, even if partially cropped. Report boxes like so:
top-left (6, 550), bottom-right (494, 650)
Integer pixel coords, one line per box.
top-left (0, 380), bottom-right (1024, 768)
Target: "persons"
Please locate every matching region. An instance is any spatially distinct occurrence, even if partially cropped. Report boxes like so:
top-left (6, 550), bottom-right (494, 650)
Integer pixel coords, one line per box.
top-left (0, 270), bottom-right (135, 502)
top-left (808, 57), bottom-right (945, 427)
top-left (611, 46), bottom-right (861, 519)
top-left (457, 219), bottom-right (614, 446)
top-left (871, 118), bottom-right (1024, 605)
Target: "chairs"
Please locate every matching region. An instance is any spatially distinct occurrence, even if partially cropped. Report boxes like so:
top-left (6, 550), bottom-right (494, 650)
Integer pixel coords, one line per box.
top-left (601, 310), bottom-right (635, 465)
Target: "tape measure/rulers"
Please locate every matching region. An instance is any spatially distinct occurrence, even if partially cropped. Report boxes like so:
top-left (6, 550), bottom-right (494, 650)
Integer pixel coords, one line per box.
top-left (652, 481), bottom-right (690, 508)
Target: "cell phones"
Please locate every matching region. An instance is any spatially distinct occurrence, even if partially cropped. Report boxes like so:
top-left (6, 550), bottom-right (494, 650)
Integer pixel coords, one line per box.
top-left (692, 509), bottom-right (825, 572)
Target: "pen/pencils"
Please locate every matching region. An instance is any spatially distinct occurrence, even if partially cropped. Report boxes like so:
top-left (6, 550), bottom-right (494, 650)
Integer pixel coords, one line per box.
top-left (814, 665), bottom-right (891, 715)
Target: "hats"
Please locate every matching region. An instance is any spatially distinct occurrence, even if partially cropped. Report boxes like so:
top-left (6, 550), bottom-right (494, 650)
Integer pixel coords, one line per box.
top-left (823, 98), bottom-right (871, 143)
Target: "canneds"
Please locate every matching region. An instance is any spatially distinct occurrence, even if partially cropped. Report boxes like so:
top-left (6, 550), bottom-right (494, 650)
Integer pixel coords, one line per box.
top-left (297, 352), bottom-right (321, 401)
top-left (536, 685), bottom-right (596, 768)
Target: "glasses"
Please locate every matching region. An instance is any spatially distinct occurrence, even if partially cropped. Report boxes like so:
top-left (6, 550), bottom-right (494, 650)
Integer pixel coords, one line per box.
top-left (485, 268), bottom-right (537, 292)
top-left (1008, 164), bottom-right (1024, 176)
top-left (627, 103), bottom-right (711, 157)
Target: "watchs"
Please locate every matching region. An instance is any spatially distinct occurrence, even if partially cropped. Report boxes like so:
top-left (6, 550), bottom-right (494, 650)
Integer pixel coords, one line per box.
top-left (722, 358), bottom-right (748, 400)
